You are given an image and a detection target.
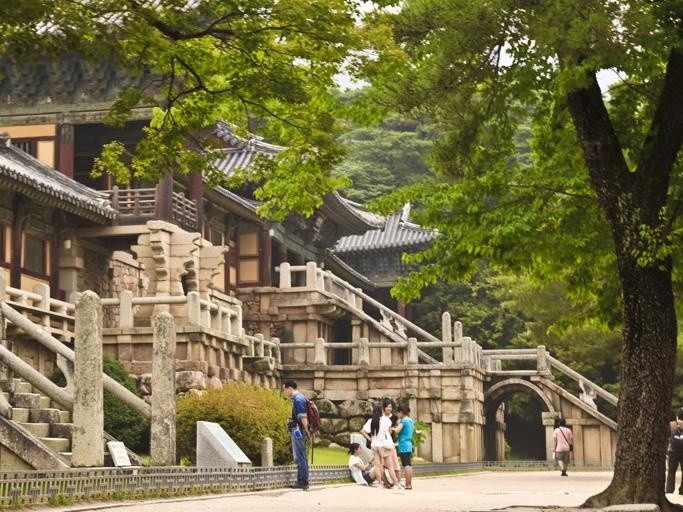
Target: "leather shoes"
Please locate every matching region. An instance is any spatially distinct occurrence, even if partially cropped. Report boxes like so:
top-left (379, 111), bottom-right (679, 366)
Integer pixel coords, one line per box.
top-left (285, 481), bottom-right (310, 491)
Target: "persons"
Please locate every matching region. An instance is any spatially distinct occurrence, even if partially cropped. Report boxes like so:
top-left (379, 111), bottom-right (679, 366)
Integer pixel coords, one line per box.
top-left (282, 380), bottom-right (315, 489)
top-left (666, 408), bottom-right (683, 495)
top-left (551, 417), bottom-right (574, 476)
top-left (348, 397), bottom-right (415, 491)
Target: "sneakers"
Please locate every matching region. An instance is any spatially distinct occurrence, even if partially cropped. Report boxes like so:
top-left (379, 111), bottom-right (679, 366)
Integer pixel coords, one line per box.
top-left (383, 480), bottom-right (413, 490)
top-left (561, 470), bottom-right (568, 476)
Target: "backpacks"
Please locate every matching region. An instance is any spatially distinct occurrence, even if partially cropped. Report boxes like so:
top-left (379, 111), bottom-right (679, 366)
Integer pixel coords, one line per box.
top-left (306, 400), bottom-right (321, 432)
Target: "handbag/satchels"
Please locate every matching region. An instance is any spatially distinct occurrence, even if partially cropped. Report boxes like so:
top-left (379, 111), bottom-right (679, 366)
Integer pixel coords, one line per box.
top-left (569, 444), bottom-right (573, 451)
